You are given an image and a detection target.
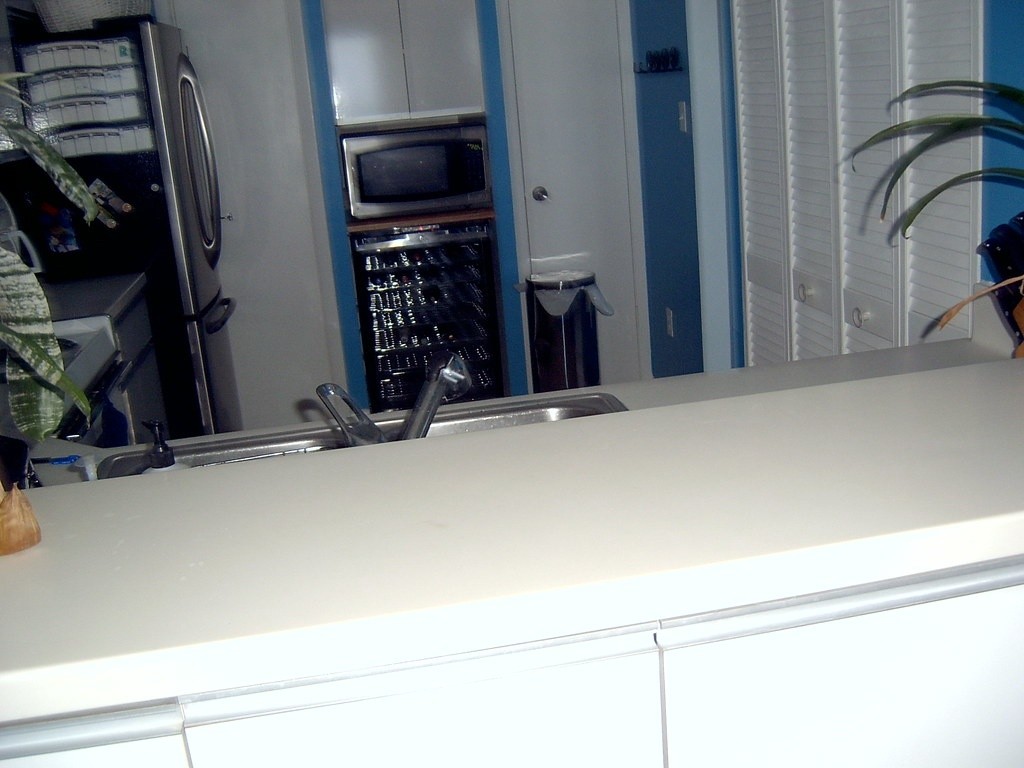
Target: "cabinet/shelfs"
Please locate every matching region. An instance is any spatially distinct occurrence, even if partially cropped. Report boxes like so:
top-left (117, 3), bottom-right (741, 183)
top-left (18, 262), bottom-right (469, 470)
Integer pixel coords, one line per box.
top-left (320, 0), bottom-right (485, 130)
top-left (0, 581), bottom-right (1024, 766)
top-left (730, 0), bottom-right (984, 369)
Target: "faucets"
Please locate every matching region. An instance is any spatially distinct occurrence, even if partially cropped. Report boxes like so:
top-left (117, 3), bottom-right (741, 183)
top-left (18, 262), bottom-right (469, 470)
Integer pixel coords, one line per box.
top-left (401, 351), bottom-right (472, 439)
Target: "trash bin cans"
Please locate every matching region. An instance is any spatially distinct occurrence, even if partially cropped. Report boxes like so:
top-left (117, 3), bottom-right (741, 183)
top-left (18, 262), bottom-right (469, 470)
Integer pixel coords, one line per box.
top-left (525, 269), bottom-right (614, 394)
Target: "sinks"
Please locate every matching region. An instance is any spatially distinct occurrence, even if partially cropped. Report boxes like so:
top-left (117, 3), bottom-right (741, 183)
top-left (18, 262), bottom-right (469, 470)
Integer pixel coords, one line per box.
top-left (92, 390), bottom-right (631, 480)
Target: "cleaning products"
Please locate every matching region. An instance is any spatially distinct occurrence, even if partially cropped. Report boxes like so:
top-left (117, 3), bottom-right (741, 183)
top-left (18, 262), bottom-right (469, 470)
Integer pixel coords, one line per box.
top-left (72, 452), bottom-right (97, 482)
top-left (138, 419), bottom-right (190, 475)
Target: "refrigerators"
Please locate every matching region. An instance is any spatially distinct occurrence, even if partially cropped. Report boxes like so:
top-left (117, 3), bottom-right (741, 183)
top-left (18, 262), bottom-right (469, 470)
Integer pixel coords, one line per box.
top-left (18, 14), bottom-right (243, 435)
